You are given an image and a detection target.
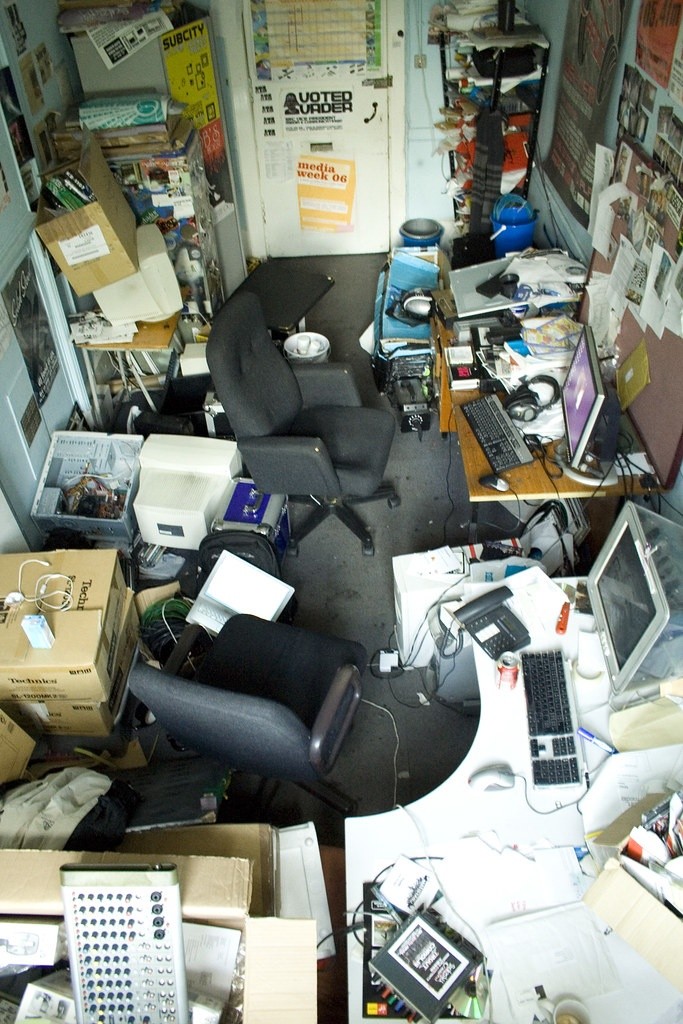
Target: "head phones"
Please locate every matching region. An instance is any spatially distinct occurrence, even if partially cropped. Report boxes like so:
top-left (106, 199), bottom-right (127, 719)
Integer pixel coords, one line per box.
top-left (503, 374), bottom-right (561, 422)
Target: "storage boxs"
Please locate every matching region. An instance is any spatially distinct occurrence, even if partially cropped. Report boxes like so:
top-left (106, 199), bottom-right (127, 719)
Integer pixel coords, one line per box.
top-left (359, 244), bottom-right (451, 395)
top-left (28, 431), bottom-right (146, 543)
top-left (0, 822), bottom-right (318, 1024)
top-left (0, 549), bottom-right (182, 786)
top-left (34, 122), bottom-right (139, 298)
top-left (581, 791), bottom-right (683, 995)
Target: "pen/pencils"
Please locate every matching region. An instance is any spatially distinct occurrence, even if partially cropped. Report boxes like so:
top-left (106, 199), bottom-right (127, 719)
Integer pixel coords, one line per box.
top-left (622, 862), bottom-right (683, 918)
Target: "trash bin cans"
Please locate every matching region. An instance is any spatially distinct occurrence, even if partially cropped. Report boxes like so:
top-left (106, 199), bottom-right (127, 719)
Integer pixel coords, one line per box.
top-left (284, 332), bottom-right (330, 364)
top-left (400, 218), bottom-right (444, 246)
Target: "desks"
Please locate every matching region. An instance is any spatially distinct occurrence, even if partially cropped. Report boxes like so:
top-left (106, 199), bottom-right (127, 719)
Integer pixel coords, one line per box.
top-left (345, 575), bottom-right (683, 1024)
top-left (430, 314), bottom-right (670, 546)
top-left (229, 264), bottom-right (335, 335)
top-left (74, 311), bottom-right (184, 431)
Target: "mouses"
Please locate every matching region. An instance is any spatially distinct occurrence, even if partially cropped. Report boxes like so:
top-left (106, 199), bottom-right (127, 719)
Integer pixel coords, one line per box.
top-left (479, 474), bottom-right (510, 492)
top-left (468, 763), bottom-right (515, 792)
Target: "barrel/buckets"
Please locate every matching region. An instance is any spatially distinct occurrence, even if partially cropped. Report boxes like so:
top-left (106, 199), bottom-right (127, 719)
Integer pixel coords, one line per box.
top-left (282, 332), bottom-right (332, 365)
top-left (489, 206), bottom-right (539, 259)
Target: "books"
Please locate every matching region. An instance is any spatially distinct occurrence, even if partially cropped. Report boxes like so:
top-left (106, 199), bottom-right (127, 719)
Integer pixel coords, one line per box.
top-left (45, 169), bottom-right (97, 204)
top-left (499, 333), bottom-right (575, 375)
top-left (367, 913), bottom-right (473, 1024)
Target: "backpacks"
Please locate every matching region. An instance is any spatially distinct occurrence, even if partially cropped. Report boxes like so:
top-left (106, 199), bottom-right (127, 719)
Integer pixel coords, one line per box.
top-left (197, 530), bottom-right (290, 624)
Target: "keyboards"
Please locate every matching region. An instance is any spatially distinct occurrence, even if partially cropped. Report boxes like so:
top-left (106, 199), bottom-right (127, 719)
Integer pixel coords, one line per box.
top-left (520, 650), bottom-right (582, 788)
top-left (461, 393), bottom-right (534, 473)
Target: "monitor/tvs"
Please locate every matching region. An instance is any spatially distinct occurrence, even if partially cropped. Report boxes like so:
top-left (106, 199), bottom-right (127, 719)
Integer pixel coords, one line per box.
top-left (92, 224), bottom-right (183, 327)
top-left (560, 326), bottom-right (619, 487)
top-left (587, 501), bottom-right (682, 696)
top-left (132, 434), bottom-right (244, 549)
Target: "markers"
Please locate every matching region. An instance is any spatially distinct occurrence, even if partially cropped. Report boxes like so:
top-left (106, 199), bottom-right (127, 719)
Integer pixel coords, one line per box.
top-left (577, 727), bottom-right (619, 755)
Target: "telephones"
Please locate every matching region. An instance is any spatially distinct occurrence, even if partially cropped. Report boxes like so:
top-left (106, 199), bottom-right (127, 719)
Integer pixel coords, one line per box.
top-left (453, 585), bottom-right (531, 661)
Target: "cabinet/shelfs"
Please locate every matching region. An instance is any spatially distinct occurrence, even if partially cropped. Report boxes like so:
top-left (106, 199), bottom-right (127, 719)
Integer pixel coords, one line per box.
top-left (439, 31), bottom-right (550, 270)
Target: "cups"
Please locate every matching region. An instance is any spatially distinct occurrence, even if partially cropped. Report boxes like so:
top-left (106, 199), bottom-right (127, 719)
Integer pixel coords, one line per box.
top-left (537, 997), bottom-right (592, 1024)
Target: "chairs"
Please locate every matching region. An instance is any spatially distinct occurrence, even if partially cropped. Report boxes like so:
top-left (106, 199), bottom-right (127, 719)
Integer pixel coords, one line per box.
top-left (128, 614), bottom-right (370, 820)
top-left (206, 290), bottom-right (401, 556)
